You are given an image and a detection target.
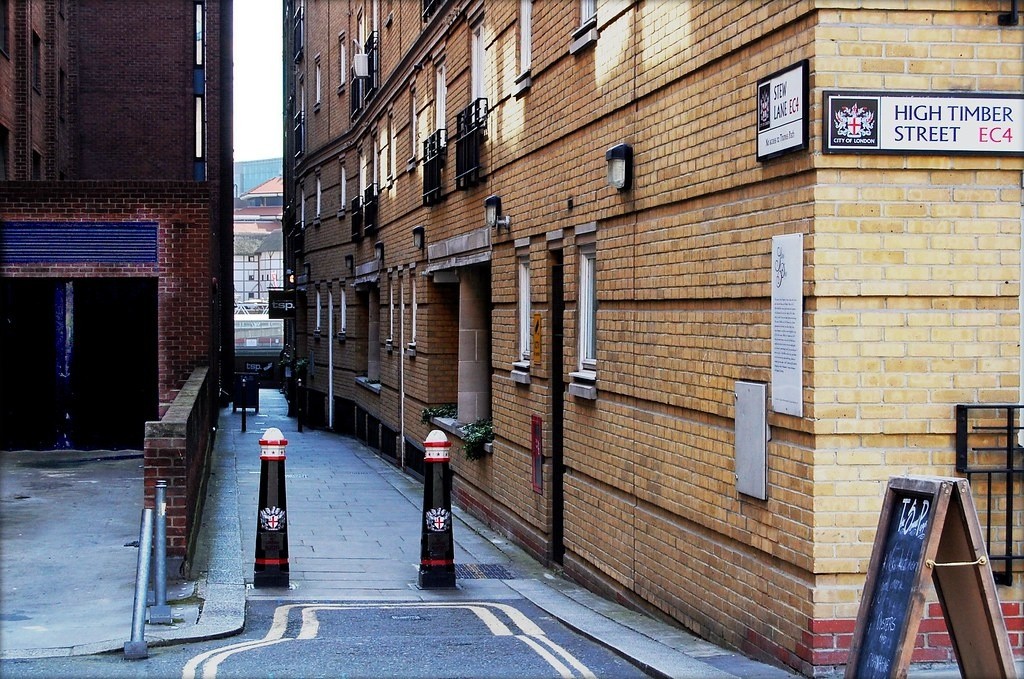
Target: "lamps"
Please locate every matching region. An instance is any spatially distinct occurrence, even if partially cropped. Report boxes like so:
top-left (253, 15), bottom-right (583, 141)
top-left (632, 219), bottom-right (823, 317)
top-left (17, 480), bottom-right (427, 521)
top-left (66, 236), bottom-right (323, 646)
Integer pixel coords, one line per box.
top-left (605, 143), bottom-right (634, 194)
top-left (374, 241), bottom-right (384, 261)
top-left (412, 226), bottom-right (425, 249)
top-left (484, 195), bottom-right (502, 227)
top-left (345, 254), bottom-right (353, 271)
top-left (304, 262), bottom-right (311, 276)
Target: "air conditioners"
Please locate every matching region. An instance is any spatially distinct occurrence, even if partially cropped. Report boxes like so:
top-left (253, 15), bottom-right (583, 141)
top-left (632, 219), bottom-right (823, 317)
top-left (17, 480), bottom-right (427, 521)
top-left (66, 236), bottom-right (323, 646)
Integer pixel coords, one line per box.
top-left (352, 54), bottom-right (368, 79)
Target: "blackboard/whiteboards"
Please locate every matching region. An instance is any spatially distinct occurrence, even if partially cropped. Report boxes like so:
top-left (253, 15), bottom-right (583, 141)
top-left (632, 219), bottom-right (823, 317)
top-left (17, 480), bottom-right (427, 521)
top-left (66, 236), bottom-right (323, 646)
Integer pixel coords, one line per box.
top-left (843, 475), bottom-right (954, 679)
top-left (234, 372), bottom-right (259, 408)
